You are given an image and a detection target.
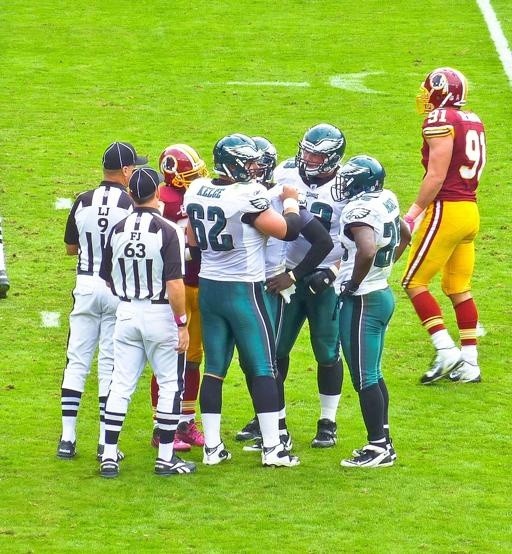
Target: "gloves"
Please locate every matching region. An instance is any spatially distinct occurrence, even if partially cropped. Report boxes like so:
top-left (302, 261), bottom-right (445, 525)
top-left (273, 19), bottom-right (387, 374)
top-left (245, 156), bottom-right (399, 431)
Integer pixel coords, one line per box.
top-left (305, 268), bottom-right (336, 295)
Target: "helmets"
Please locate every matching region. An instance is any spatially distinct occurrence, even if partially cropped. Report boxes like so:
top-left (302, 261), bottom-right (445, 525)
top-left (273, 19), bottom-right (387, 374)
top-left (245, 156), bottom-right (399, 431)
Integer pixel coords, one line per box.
top-left (159, 144), bottom-right (210, 192)
top-left (213, 133), bottom-right (277, 183)
top-left (416, 67), bottom-right (468, 115)
top-left (331, 155), bottom-right (386, 201)
top-left (295, 123), bottom-right (346, 178)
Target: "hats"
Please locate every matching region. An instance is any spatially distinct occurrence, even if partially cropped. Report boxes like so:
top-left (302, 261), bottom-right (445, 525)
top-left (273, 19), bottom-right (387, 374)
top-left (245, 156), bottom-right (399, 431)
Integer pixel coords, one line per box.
top-left (129, 166), bottom-right (165, 199)
top-left (102, 142), bottom-right (148, 169)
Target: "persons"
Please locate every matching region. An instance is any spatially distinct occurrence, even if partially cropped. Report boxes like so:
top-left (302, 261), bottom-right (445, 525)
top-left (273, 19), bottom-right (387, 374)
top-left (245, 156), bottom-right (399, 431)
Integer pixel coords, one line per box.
top-left (402, 66), bottom-right (486, 385)
top-left (57, 122), bottom-right (411, 477)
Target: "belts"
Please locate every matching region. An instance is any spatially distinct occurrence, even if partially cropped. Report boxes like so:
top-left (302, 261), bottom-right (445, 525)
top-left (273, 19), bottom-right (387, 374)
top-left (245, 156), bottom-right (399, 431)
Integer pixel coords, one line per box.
top-left (121, 298), bottom-right (169, 304)
top-left (77, 270), bottom-right (93, 275)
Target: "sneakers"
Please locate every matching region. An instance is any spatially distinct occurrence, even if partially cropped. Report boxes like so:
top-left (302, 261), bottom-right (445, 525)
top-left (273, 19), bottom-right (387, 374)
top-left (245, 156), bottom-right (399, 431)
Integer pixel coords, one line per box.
top-left (151, 419), bottom-right (231, 475)
top-left (311, 418), bottom-right (337, 448)
top-left (236, 419), bottom-right (300, 468)
top-left (340, 443), bottom-right (396, 468)
top-left (97, 444), bottom-right (124, 478)
top-left (444, 360), bottom-right (481, 383)
top-left (421, 346), bottom-right (465, 384)
top-left (0, 270), bottom-right (10, 298)
top-left (57, 435), bottom-right (76, 460)
